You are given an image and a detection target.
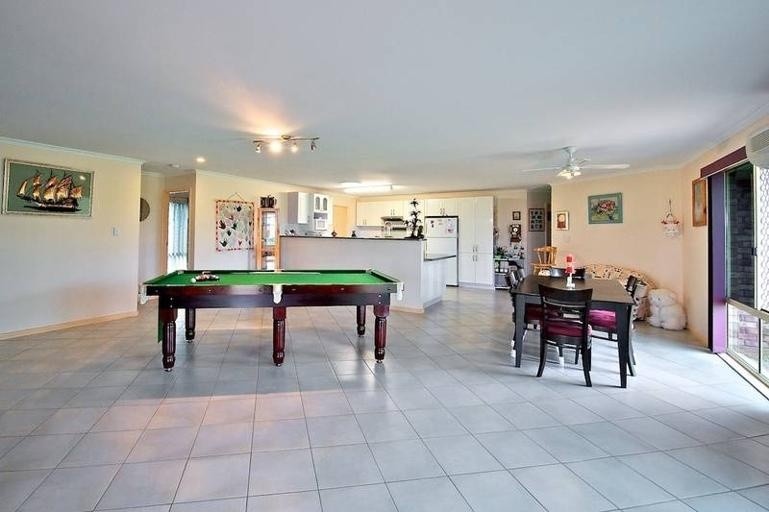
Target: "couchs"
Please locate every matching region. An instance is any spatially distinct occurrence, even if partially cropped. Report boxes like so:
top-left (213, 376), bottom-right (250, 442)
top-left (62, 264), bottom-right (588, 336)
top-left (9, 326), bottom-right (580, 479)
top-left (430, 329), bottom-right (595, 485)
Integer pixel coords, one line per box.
top-left (573, 263), bottom-right (655, 329)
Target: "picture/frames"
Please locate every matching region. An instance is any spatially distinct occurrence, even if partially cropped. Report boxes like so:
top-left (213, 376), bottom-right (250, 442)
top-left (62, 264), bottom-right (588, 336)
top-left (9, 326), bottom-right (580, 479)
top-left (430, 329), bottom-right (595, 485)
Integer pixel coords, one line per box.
top-left (1, 158), bottom-right (97, 219)
top-left (691, 178), bottom-right (706, 226)
top-left (588, 193), bottom-right (623, 224)
top-left (528, 208), bottom-right (545, 232)
top-left (555, 211), bottom-right (568, 230)
top-left (215, 199), bottom-right (254, 253)
top-left (512, 211), bottom-right (521, 220)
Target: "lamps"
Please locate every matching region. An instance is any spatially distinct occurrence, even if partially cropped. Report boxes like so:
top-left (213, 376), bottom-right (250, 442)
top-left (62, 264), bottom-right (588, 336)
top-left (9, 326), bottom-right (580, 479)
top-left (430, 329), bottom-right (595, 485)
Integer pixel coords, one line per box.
top-left (255, 140), bottom-right (320, 152)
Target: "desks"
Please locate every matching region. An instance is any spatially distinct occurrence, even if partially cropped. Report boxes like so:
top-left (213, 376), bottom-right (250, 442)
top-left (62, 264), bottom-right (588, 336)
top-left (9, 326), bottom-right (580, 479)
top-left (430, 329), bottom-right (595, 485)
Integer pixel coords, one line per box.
top-left (142, 268), bottom-right (405, 372)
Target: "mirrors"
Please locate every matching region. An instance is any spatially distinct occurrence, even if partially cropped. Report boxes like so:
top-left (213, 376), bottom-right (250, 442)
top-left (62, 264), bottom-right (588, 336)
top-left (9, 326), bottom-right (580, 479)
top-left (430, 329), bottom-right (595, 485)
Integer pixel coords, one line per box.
top-left (255, 207), bottom-right (280, 270)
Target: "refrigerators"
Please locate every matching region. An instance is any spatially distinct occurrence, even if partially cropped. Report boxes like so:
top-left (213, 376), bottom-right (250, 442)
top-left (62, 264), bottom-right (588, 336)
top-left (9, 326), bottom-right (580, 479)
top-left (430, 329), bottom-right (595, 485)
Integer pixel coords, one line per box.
top-left (425, 217), bottom-right (458, 286)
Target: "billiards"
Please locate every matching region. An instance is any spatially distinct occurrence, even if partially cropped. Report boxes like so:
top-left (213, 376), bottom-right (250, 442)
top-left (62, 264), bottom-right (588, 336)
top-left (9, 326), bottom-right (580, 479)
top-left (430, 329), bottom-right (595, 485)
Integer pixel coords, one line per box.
top-left (191, 278), bottom-right (196, 283)
top-left (196, 274), bottom-right (220, 280)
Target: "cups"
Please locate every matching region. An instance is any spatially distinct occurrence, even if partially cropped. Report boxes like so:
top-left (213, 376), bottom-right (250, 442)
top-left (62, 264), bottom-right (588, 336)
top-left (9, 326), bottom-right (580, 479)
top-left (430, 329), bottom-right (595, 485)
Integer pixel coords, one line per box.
top-left (384, 221), bottom-right (393, 237)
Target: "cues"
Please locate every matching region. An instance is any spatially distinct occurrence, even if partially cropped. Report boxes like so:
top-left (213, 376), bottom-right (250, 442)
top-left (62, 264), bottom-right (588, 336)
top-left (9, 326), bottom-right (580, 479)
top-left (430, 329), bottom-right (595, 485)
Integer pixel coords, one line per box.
top-left (233, 271), bottom-right (321, 275)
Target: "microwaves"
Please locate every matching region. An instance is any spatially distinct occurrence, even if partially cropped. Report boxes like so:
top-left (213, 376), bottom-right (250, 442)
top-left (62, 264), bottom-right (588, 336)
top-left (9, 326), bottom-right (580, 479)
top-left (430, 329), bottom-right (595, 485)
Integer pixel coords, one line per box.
top-left (314, 218), bottom-right (327, 229)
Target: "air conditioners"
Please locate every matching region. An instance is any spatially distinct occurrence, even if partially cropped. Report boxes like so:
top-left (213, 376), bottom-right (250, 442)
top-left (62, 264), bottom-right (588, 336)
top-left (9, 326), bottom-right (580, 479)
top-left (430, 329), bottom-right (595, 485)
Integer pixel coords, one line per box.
top-left (745, 125), bottom-right (769, 169)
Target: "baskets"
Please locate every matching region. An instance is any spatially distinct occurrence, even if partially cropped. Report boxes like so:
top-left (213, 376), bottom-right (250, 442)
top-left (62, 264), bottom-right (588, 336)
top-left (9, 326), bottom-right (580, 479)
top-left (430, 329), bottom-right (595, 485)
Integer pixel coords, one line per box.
top-left (664, 212), bottom-right (680, 237)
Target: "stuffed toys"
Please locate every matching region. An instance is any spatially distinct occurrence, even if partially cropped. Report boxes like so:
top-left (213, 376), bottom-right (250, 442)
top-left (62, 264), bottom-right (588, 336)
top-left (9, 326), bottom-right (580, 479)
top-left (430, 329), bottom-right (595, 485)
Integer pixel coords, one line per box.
top-left (657, 200), bottom-right (680, 238)
top-left (645, 288), bottom-right (687, 331)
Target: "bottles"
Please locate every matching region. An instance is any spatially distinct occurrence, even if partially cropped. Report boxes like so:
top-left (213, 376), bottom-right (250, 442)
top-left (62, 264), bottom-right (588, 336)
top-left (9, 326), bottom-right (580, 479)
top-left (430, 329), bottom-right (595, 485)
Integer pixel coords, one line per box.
top-left (513, 246), bottom-right (518, 258)
top-left (521, 247), bottom-right (525, 259)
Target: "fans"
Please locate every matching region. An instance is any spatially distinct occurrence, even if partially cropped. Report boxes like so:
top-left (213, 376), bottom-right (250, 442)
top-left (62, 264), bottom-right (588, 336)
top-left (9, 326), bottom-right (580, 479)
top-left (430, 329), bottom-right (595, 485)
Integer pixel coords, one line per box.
top-left (519, 146), bottom-right (629, 179)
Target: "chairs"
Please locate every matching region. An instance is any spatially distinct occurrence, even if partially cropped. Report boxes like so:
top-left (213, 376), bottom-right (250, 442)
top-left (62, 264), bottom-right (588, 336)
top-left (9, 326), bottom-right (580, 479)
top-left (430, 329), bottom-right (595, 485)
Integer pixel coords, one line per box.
top-left (504, 267), bottom-right (650, 388)
top-left (532, 245), bottom-right (557, 275)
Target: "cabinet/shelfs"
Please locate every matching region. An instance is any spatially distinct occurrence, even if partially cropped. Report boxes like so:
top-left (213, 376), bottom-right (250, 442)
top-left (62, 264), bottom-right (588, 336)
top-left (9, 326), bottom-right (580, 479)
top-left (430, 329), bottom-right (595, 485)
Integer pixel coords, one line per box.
top-left (494, 258), bottom-right (523, 290)
top-left (310, 193), bottom-right (330, 232)
top-left (458, 195), bottom-right (493, 288)
top-left (288, 191), bottom-right (309, 224)
top-left (356, 199), bottom-right (425, 229)
top-left (424, 198), bottom-right (458, 217)
